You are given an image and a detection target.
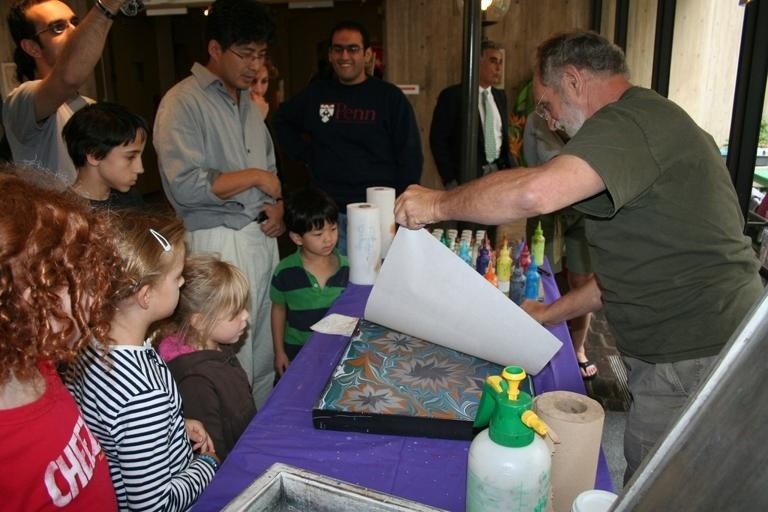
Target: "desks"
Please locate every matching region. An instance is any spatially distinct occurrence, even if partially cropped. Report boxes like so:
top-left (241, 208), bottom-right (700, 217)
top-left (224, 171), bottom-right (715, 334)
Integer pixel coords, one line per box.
top-left (186, 250), bottom-right (616, 512)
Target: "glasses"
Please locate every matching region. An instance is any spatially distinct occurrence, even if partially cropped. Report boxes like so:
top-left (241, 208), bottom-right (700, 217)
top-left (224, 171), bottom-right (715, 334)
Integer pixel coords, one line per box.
top-left (332, 44), bottom-right (366, 55)
top-left (535, 90), bottom-right (548, 121)
top-left (229, 49), bottom-right (270, 66)
top-left (35, 18), bottom-right (80, 38)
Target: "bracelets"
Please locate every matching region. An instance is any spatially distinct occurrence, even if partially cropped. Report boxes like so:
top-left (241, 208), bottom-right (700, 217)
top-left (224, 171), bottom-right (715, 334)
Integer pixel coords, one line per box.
top-left (95, 0), bottom-right (118, 20)
top-left (194, 455), bottom-right (219, 471)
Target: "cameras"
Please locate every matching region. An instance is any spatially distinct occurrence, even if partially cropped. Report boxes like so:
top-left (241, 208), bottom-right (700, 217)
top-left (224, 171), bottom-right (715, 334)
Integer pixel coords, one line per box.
top-left (120, 0), bottom-right (147, 19)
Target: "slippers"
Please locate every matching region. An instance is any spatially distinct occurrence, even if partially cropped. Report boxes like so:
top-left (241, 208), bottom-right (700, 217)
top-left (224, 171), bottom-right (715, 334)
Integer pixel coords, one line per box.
top-left (578, 360), bottom-right (597, 380)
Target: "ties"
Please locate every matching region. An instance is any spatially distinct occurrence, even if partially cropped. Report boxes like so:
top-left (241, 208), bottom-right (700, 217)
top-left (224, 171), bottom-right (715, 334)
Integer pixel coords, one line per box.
top-left (481, 90), bottom-right (497, 164)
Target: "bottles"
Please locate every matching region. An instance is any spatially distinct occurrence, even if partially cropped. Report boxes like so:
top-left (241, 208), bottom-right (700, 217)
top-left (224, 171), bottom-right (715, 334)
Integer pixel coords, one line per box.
top-left (459, 221), bottom-right (546, 306)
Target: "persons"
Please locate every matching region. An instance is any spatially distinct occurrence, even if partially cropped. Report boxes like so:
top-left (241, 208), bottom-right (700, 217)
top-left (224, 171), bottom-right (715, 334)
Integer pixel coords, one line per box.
top-left (152, 254), bottom-right (258, 467)
top-left (0, 0), bottom-right (129, 190)
top-left (270, 20), bottom-right (425, 254)
top-left (427, 38), bottom-right (517, 237)
top-left (249, 58), bottom-right (282, 123)
top-left (267, 178), bottom-right (350, 389)
top-left (64, 204), bottom-right (220, 511)
top-left (62, 103), bottom-right (149, 210)
top-left (151, 0), bottom-right (290, 411)
top-left (394, 28), bottom-right (766, 490)
top-left (522, 109), bottom-right (598, 382)
top-left (1, 167), bottom-right (136, 512)
